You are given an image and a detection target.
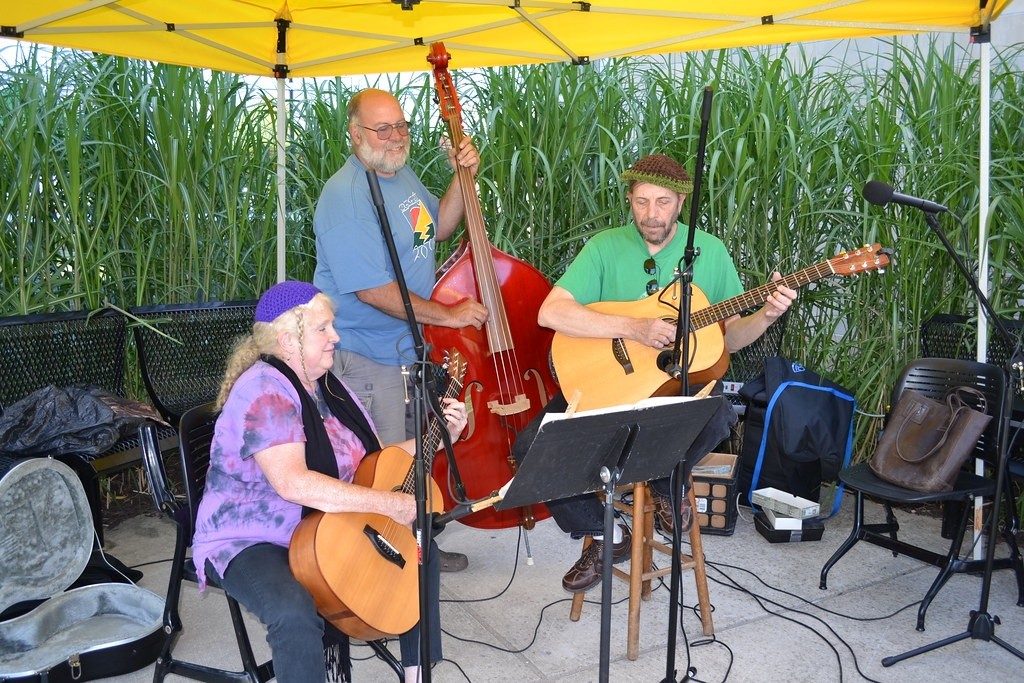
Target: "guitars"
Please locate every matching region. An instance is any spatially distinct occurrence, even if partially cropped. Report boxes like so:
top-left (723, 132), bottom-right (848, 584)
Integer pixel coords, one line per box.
top-left (287, 346), bottom-right (468, 641)
top-left (551, 242), bottom-right (889, 414)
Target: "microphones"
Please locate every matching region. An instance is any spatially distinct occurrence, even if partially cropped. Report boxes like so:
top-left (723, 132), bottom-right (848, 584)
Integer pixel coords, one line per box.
top-left (862, 181), bottom-right (948, 213)
top-left (412, 502), bottom-right (474, 540)
top-left (656, 350), bottom-right (683, 380)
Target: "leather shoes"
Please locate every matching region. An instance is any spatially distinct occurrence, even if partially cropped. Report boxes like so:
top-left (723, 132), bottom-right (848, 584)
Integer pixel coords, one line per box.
top-left (648, 483), bottom-right (694, 536)
top-left (562, 524), bottom-right (633, 592)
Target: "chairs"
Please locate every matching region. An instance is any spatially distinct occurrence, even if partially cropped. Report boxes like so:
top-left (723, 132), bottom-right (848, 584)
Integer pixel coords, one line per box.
top-left (0, 299), bottom-right (1024, 683)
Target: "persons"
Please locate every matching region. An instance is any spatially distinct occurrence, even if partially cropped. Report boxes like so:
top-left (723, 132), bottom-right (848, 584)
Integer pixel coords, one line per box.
top-left (313, 89), bottom-right (491, 573)
top-left (539, 154), bottom-right (797, 592)
top-left (193, 282), bottom-right (468, 683)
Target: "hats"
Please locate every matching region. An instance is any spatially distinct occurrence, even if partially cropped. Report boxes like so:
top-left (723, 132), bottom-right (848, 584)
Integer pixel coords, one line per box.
top-left (254, 281), bottom-right (321, 322)
top-left (620, 154), bottom-right (694, 193)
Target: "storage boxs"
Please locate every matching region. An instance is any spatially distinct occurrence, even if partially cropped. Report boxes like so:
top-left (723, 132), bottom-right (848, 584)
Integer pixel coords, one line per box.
top-left (690, 465), bottom-right (743, 536)
top-left (690, 452), bottom-right (739, 528)
top-left (753, 514), bottom-right (824, 543)
top-left (752, 485), bottom-right (824, 520)
top-left (762, 506), bottom-right (803, 530)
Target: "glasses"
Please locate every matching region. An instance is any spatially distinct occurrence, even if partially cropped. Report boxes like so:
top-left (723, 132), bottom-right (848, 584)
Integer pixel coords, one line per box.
top-left (356, 121), bottom-right (412, 141)
top-left (643, 256), bottom-right (659, 296)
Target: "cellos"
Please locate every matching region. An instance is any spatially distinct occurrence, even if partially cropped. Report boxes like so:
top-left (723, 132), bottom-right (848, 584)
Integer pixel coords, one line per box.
top-left (422, 41), bottom-right (562, 565)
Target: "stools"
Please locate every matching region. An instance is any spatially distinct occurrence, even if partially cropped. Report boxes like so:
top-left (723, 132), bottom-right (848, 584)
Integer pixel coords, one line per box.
top-left (569, 474), bottom-right (715, 662)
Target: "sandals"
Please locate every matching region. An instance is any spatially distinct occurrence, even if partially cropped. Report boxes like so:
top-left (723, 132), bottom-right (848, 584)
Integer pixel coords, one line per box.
top-left (437, 548), bottom-right (468, 572)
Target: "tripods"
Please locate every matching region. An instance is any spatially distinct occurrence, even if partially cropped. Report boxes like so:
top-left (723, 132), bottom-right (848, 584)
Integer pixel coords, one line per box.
top-left (881, 210), bottom-right (1024, 670)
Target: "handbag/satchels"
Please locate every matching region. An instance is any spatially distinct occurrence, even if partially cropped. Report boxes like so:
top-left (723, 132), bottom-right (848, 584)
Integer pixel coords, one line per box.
top-left (736, 357), bottom-right (857, 522)
top-left (869, 386), bottom-right (993, 492)
